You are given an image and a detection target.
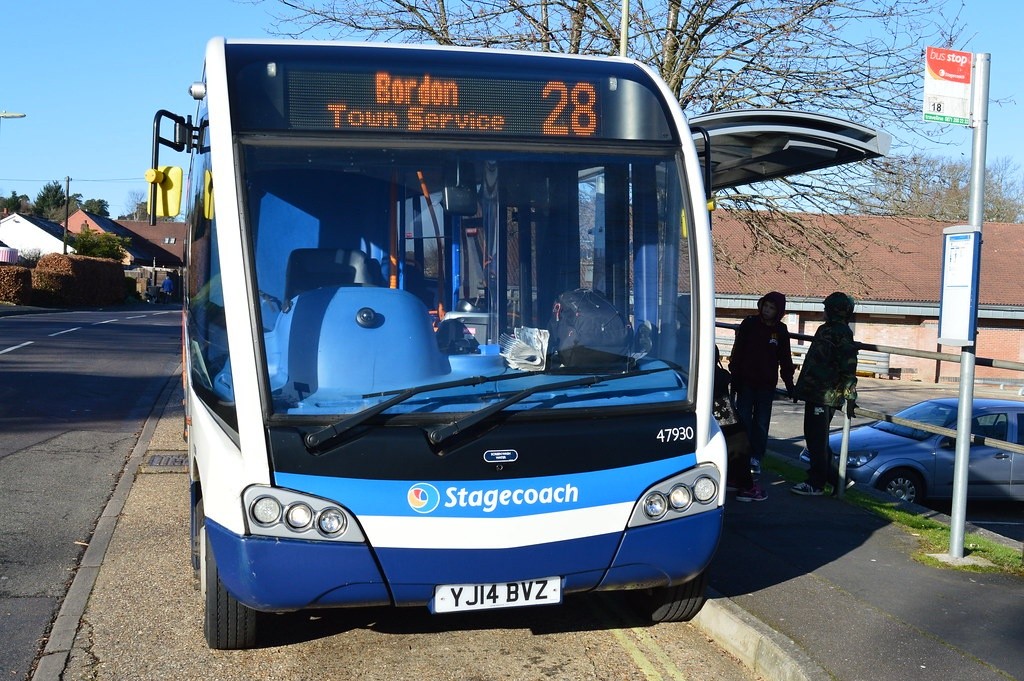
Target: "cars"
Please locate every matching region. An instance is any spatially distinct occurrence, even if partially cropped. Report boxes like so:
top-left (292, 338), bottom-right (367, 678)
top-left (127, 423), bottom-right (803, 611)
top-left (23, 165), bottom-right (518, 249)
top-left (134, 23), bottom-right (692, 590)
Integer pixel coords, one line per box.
top-left (798, 399), bottom-right (1024, 505)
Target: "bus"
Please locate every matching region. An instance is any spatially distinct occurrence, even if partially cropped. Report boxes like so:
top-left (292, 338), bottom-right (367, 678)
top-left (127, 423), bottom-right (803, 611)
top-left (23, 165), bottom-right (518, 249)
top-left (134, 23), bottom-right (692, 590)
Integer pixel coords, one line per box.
top-left (144, 38), bottom-right (727, 649)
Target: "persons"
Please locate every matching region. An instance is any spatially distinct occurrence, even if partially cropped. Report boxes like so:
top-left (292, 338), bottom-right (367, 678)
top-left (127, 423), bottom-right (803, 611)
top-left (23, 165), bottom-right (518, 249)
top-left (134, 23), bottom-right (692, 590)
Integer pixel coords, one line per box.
top-left (792, 292), bottom-right (858, 495)
top-left (712, 346), bottom-right (768, 502)
top-left (729, 292), bottom-right (795, 479)
top-left (162, 276), bottom-right (174, 306)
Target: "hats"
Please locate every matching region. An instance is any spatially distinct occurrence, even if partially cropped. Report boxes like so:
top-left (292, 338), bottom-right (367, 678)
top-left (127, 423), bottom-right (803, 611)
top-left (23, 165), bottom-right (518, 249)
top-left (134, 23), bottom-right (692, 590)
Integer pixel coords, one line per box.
top-left (822, 292), bottom-right (853, 317)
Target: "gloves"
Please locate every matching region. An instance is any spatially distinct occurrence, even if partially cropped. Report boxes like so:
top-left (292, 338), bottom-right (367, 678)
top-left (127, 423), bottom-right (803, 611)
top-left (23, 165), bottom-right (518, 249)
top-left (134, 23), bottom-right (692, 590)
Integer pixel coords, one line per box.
top-left (847, 399), bottom-right (859, 418)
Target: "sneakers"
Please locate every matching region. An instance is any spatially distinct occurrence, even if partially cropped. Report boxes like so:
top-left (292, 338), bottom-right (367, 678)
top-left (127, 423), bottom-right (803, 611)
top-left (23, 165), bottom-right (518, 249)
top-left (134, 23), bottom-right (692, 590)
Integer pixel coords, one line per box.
top-left (735, 488), bottom-right (768, 502)
top-left (749, 457), bottom-right (761, 474)
top-left (790, 483), bottom-right (824, 495)
top-left (726, 481), bottom-right (764, 492)
top-left (830, 478), bottom-right (856, 498)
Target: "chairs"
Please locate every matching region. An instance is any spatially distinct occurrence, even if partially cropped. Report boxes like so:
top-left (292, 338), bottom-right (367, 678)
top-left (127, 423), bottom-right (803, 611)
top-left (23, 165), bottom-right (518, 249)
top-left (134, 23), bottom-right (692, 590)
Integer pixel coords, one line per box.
top-left (283, 247), bottom-right (373, 303)
